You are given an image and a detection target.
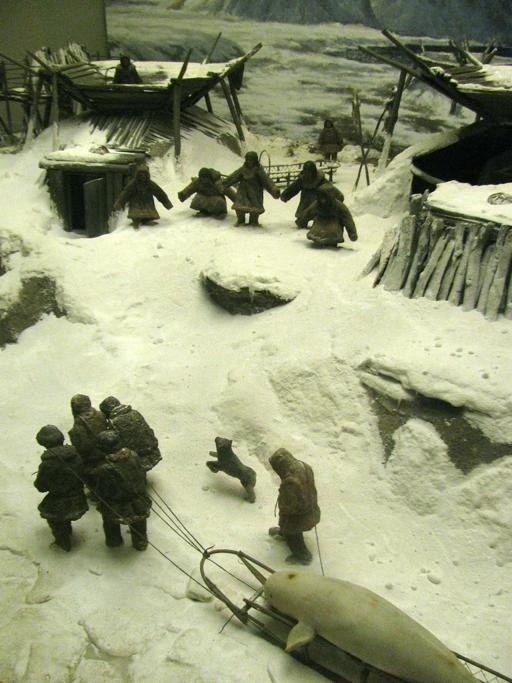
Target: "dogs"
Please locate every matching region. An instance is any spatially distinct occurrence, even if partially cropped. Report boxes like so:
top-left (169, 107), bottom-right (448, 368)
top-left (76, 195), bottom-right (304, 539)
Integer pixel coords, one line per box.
top-left (206, 436), bottom-right (257, 504)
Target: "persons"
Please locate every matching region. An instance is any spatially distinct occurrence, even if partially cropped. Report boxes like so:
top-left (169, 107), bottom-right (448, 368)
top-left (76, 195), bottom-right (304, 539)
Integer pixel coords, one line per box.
top-left (318, 118), bottom-right (343, 162)
top-left (111, 164), bottom-right (174, 229)
top-left (90, 428), bottom-right (154, 551)
top-left (266, 445), bottom-right (322, 568)
top-left (295, 181), bottom-right (359, 248)
top-left (178, 165), bottom-right (238, 219)
top-left (66, 392), bottom-right (108, 502)
top-left (98, 395), bottom-right (163, 486)
top-left (31, 423), bottom-right (90, 552)
top-left (111, 52), bottom-right (142, 83)
top-left (216, 150), bottom-right (281, 226)
top-left (279, 159), bottom-right (345, 228)
